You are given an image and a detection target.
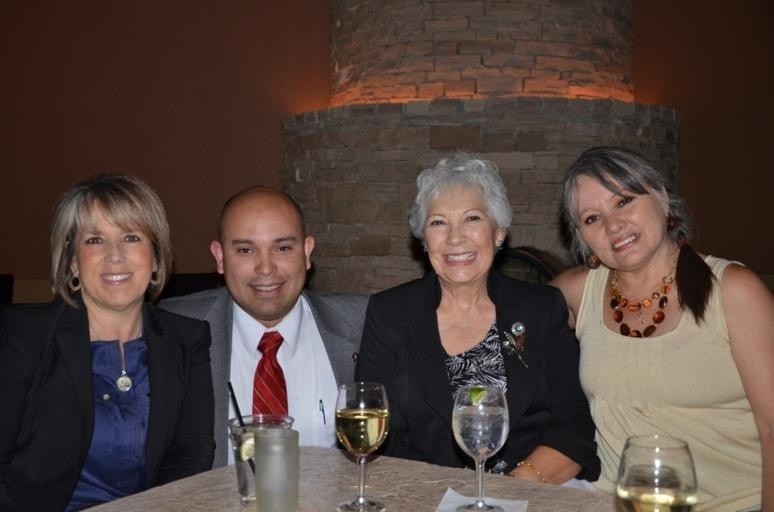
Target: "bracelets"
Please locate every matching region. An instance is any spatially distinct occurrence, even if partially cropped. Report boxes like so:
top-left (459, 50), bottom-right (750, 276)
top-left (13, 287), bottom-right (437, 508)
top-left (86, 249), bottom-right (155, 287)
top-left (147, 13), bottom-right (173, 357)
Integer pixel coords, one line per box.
top-left (516, 460), bottom-right (544, 481)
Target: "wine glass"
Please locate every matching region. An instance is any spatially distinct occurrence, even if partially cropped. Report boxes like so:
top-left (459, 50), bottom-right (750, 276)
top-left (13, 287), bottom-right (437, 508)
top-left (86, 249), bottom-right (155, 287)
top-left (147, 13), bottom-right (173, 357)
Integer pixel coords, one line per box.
top-left (333, 380), bottom-right (390, 511)
top-left (451, 384), bottom-right (510, 512)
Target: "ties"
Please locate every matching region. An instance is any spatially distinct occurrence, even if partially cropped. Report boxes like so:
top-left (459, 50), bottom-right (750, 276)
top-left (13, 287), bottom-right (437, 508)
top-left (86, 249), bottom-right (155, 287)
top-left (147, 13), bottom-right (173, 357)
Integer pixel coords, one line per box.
top-left (253, 332), bottom-right (289, 424)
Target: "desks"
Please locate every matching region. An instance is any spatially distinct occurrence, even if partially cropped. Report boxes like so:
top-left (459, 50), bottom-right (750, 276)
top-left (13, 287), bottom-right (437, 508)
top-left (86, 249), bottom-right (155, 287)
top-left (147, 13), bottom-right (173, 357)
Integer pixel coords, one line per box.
top-left (78, 446), bottom-right (618, 512)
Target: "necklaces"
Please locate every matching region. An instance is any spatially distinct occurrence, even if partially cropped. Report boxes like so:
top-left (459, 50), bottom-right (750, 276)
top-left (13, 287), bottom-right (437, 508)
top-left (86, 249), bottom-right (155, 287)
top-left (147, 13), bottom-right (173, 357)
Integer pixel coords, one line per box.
top-left (609, 243), bottom-right (678, 337)
top-left (88, 321), bottom-right (141, 391)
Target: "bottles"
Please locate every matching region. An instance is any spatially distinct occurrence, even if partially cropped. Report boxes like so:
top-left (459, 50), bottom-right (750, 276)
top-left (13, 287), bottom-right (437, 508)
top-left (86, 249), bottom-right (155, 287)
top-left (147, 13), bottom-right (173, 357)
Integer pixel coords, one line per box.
top-left (227, 413), bottom-right (301, 512)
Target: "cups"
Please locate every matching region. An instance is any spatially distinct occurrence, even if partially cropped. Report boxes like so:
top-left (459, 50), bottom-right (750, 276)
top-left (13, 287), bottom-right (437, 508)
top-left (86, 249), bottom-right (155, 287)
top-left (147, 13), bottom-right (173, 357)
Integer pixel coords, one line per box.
top-left (614, 433), bottom-right (698, 512)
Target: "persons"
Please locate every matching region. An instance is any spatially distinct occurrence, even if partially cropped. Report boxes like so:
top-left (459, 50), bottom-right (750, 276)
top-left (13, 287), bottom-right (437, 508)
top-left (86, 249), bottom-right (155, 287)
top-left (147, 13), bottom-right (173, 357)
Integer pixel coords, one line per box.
top-left (353, 151), bottom-right (601, 491)
top-left (544, 147), bottom-right (773, 511)
top-left (154, 187), bottom-right (370, 472)
top-left (0, 172), bottom-right (214, 509)
